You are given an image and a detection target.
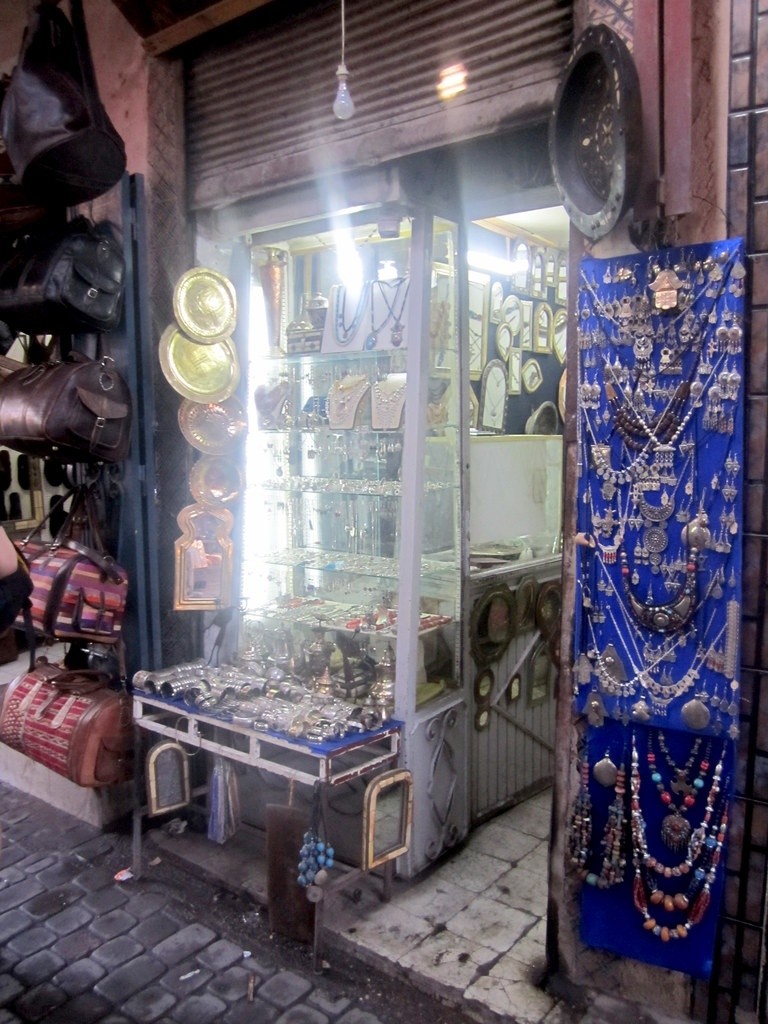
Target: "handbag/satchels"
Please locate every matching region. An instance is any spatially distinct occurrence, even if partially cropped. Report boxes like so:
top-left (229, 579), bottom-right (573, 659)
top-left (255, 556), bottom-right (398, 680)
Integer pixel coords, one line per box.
top-left (0, 0), bottom-right (127, 647)
top-left (1, 656), bottom-right (132, 787)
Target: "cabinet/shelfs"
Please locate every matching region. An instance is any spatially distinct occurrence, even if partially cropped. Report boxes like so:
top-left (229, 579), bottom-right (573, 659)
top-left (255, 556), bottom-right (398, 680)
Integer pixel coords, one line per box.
top-left (129, 192), bottom-right (466, 972)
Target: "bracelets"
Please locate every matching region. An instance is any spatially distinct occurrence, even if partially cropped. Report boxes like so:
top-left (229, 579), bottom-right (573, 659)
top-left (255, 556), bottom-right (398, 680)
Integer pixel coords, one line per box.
top-left (132, 657), bottom-right (382, 744)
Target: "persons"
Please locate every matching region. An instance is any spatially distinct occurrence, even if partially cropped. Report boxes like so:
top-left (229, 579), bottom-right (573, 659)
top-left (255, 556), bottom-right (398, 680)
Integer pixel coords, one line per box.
top-left (0, 523), bottom-right (34, 634)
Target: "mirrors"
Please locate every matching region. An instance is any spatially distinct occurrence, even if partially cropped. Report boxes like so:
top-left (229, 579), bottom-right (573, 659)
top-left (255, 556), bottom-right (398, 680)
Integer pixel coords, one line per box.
top-left (431, 240), bottom-right (566, 431)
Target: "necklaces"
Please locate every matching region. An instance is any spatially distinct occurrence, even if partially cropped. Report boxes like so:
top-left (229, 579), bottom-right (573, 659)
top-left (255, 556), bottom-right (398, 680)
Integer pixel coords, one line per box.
top-left (259, 247), bottom-right (512, 636)
top-left (563, 245), bottom-right (742, 942)
